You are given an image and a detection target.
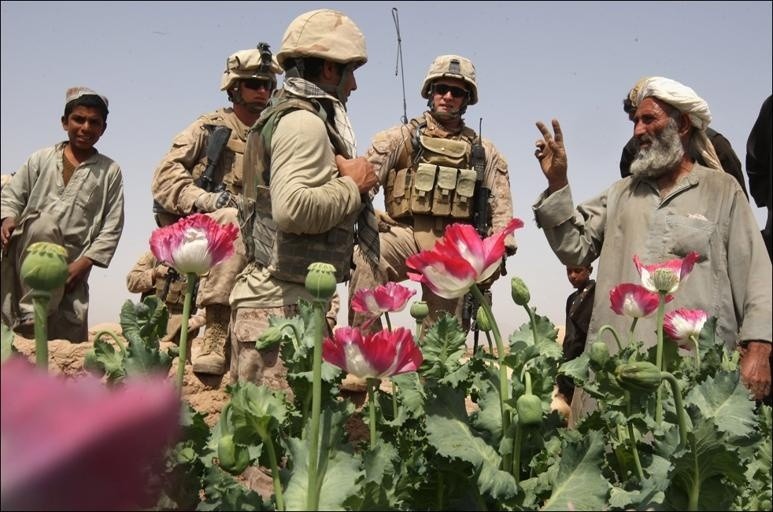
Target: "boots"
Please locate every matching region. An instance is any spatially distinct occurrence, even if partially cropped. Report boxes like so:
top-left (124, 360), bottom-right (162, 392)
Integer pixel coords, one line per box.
top-left (192, 302), bottom-right (232, 377)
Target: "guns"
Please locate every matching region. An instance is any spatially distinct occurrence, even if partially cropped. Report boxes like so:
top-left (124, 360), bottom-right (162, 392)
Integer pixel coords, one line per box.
top-left (160, 125), bottom-right (231, 300)
top-left (464, 187), bottom-right (489, 355)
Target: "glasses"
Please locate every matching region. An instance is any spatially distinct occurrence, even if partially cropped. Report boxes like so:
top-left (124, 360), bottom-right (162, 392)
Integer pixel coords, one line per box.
top-left (237, 78), bottom-right (272, 91)
top-left (429, 83), bottom-right (469, 98)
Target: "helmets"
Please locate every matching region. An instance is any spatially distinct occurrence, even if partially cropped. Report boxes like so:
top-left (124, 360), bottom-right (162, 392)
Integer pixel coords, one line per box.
top-left (420, 55), bottom-right (478, 105)
top-left (276, 8), bottom-right (368, 69)
top-left (220, 49), bottom-right (283, 91)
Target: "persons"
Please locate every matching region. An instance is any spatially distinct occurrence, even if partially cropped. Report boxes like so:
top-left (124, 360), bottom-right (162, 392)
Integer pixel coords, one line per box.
top-left (126, 249), bottom-right (207, 359)
top-left (532, 76), bottom-right (772, 432)
top-left (152, 48), bottom-right (284, 376)
top-left (225, 9), bottom-right (378, 502)
top-left (338, 54), bottom-right (517, 393)
top-left (620, 95), bottom-right (749, 201)
top-left (746, 95), bottom-right (773, 263)
top-left (0, 87), bottom-right (125, 344)
top-left (562, 263), bottom-right (596, 360)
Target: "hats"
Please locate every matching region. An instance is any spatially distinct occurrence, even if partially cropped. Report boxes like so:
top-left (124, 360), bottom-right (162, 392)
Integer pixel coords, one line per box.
top-left (65, 86), bottom-right (110, 114)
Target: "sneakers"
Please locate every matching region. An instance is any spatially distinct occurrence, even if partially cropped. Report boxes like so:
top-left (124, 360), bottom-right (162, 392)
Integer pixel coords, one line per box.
top-left (339, 374), bottom-right (368, 392)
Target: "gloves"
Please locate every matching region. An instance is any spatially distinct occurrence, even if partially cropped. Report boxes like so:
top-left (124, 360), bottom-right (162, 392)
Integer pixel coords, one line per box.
top-left (154, 264), bottom-right (180, 283)
top-left (196, 190), bottom-right (240, 214)
